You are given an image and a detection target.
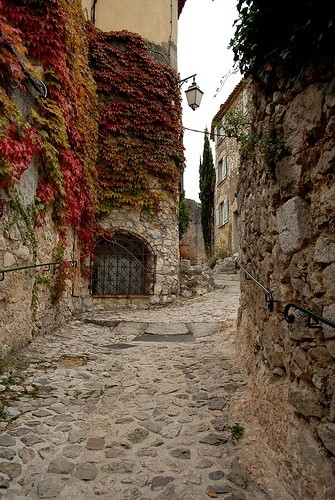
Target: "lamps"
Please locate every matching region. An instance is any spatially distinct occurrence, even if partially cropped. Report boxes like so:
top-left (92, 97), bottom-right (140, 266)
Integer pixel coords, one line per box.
top-left (178, 73), bottom-right (204, 111)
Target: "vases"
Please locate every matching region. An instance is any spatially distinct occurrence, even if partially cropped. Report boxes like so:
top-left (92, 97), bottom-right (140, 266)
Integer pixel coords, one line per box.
top-left (179, 244), bottom-right (189, 259)
